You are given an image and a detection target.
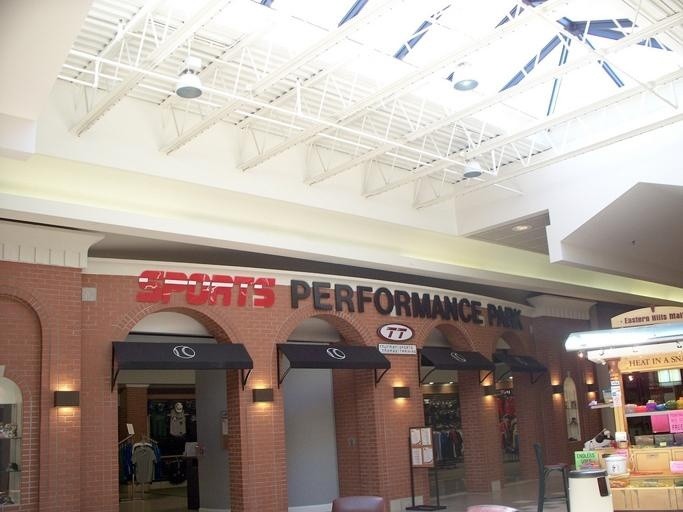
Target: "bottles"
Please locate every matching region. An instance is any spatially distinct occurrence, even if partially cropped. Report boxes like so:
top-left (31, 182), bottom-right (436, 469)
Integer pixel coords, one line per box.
top-left (656, 400), bottom-right (683, 411)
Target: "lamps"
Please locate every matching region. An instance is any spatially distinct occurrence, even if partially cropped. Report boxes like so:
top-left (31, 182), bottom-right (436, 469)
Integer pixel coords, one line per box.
top-left (484, 386), bottom-right (495, 396)
top-left (657, 369), bottom-right (682, 387)
top-left (252, 388), bottom-right (273, 402)
top-left (54, 390), bottom-right (80, 407)
top-left (445, 62), bottom-right (479, 91)
top-left (463, 156), bottom-right (481, 178)
top-left (175, 58), bottom-right (203, 99)
top-left (393, 386), bottom-right (410, 399)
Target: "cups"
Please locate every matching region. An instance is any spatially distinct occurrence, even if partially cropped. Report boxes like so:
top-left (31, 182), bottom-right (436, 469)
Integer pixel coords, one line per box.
top-left (646, 402), bottom-right (657, 412)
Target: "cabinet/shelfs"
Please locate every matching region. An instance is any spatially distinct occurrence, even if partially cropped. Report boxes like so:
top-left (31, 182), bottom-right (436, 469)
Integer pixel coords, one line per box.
top-left (0, 436), bottom-right (20, 506)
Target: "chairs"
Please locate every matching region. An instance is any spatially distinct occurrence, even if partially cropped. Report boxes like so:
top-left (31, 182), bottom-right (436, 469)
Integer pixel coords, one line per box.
top-left (466, 505), bottom-right (520, 512)
top-left (332, 496), bottom-right (385, 512)
top-left (533, 443), bottom-right (569, 512)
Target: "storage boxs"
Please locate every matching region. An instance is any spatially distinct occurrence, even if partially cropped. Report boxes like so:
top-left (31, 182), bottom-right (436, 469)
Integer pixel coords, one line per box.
top-left (634, 433), bottom-right (683, 446)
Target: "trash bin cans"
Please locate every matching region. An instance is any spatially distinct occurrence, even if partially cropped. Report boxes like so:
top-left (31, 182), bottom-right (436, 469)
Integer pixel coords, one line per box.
top-left (567, 469), bottom-right (614, 512)
